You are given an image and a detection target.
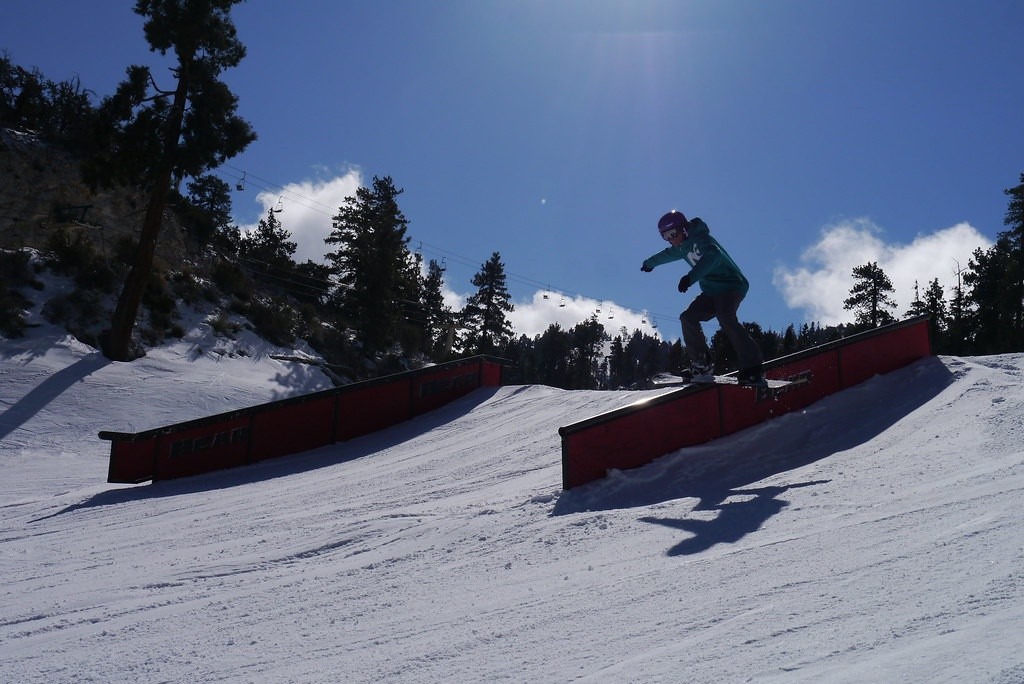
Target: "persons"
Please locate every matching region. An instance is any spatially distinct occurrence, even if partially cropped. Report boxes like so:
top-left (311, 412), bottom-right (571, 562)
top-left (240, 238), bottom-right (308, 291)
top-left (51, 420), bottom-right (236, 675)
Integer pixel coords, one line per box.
top-left (640, 211), bottom-right (769, 388)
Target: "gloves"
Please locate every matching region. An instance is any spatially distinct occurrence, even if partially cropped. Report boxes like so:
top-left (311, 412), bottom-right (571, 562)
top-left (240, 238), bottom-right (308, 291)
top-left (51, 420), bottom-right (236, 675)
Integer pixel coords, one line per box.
top-left (678, 282), bottom-right (688, 293)
top-left (640, 264), bottom-right (654, 272)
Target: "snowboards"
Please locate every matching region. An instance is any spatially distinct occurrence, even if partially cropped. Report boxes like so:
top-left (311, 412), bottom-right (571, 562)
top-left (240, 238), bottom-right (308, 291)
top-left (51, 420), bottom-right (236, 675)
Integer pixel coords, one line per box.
top-left (652, 372), bottom-right (808, 389)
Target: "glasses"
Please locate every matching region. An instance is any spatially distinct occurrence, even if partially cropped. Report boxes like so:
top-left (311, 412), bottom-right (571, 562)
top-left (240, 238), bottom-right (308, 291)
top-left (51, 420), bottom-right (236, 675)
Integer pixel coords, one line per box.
top-left (661, 228), bottom-right (679, 241)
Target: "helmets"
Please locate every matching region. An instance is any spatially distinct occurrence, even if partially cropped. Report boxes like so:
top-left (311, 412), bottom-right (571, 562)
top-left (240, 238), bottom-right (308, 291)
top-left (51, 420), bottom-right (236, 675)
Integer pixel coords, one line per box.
top-left (658, 211), bottom-right (688, 233)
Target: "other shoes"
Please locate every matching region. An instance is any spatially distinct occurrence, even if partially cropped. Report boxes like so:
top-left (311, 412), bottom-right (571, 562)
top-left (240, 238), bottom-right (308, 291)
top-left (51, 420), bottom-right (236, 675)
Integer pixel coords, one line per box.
top-left (679, 362), bottom-right (714, 377)
top-left (738, 364), bottom-right (764, 385)
